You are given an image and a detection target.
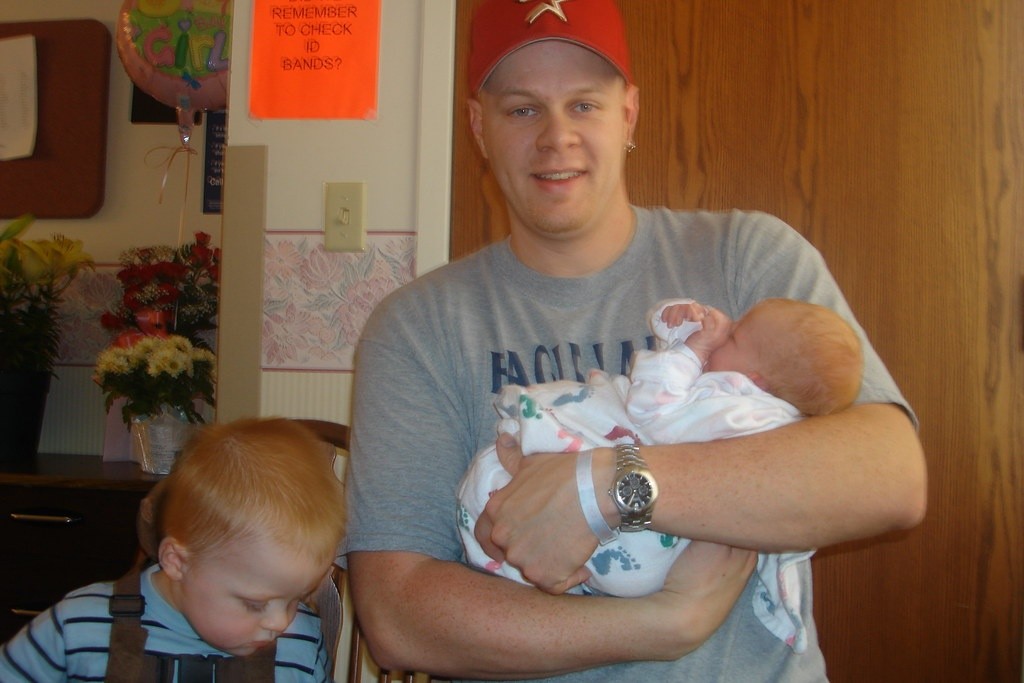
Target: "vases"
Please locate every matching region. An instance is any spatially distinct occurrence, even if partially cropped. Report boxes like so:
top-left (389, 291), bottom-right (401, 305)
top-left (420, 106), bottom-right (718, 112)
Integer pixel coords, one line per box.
top-left (0, 395), bottom-right (44, 474)
top-left (130, 406), bottom-right (197, 475)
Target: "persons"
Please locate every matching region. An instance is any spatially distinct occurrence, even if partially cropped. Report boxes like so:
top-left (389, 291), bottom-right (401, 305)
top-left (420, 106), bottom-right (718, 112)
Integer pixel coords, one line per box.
top-left (459, 296), bottom-right (866, 597)
top-left (0, 415), bottom-right (346, 683)
top-left (333, 0), bottom-right (930, 683)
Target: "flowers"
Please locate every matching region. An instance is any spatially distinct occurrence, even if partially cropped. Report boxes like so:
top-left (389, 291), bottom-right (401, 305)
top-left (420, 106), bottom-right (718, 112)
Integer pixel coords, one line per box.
top-left (0, 218), bottom-right (98, 394)
top-left (93, 229), bottom-right (225, 425)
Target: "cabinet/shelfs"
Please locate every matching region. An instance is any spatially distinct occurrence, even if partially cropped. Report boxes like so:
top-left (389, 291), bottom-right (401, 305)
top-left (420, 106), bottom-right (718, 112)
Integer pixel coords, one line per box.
top-left (0, 454), bottom-right (152, 641)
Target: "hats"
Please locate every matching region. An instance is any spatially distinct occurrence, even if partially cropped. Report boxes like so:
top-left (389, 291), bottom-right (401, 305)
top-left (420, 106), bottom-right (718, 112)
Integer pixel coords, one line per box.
top-left (470, 0), bottom-right (634, 102)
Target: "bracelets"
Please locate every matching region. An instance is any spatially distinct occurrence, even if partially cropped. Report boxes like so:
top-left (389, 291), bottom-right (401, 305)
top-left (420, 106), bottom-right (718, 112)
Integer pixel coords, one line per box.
top-left (576, 449), bottom-right (621, 545)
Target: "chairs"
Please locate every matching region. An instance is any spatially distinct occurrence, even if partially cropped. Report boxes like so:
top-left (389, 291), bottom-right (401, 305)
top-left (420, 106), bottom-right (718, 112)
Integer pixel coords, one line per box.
top-left (139, 419), bottom-right (364, 683)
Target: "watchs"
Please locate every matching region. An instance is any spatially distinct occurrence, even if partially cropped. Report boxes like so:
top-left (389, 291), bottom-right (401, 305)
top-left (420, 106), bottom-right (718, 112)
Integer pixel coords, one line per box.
top-left (607, 443), bottom-right (660, 530)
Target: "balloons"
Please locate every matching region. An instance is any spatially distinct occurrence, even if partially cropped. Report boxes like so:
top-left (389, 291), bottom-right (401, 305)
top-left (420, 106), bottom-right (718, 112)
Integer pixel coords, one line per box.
top-left (116, 0), bottom-right (232, 148)
